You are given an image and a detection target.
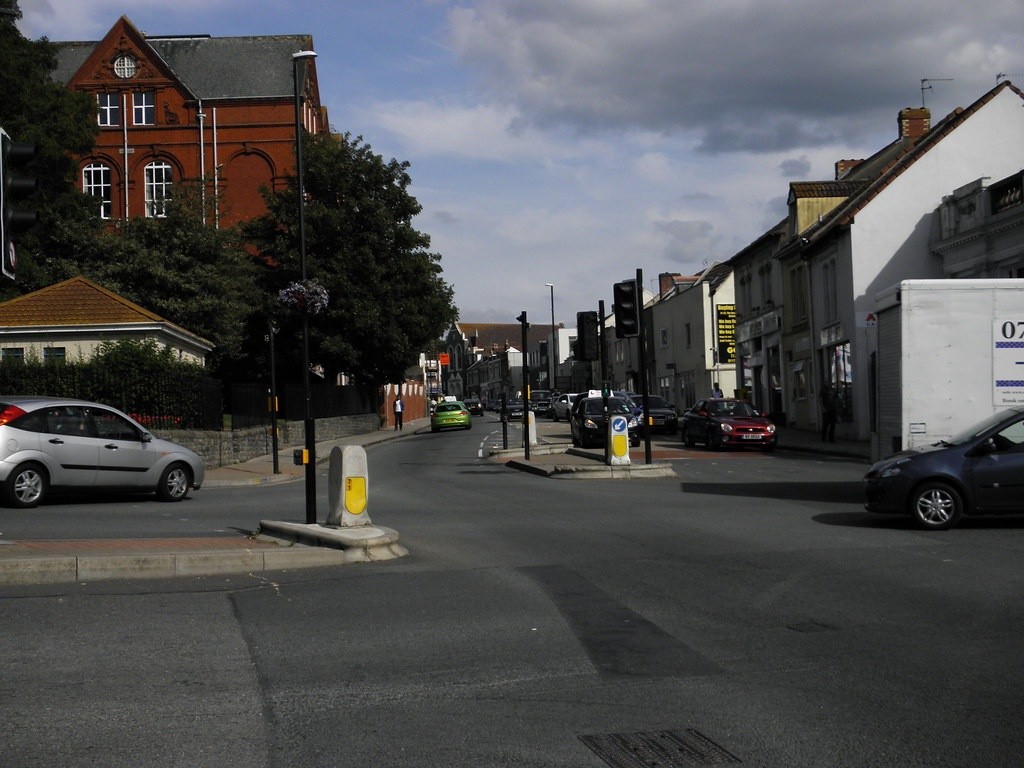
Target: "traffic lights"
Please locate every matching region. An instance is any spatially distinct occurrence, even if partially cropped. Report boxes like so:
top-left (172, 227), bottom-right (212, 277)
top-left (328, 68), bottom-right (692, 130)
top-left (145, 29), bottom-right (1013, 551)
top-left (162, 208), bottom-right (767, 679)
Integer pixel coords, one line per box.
top-left (576, 311), bottom-right (599, 363)
top-left (613, 278), bottom-right (640, 340)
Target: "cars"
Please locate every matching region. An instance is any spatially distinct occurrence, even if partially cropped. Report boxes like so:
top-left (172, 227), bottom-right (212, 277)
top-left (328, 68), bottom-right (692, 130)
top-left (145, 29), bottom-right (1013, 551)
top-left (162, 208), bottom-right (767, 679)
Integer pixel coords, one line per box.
top-left (551, 394), bottom-right (579, 423)
top-left (682, 398), bottom-right (778, 453)
top-left (862, 406), bottom-right (1024, 529)
top-left (499, 397), bottom-right (531, 422)
top-left (463, 399), bottom-right (484, 416)
top-left (569, 390), bottom-right (640, 447)
top-left (628, 395), bottom-right (678, 436)
top-left (431, 401), bottom-right (472, 433)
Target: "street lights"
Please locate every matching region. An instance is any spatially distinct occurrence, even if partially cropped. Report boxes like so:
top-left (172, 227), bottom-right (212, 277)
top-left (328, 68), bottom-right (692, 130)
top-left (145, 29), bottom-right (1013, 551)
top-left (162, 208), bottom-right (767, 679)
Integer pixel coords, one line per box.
top-left (545, 284), bottom-right (557, 389)
top-left (293, 49), bottom-right (319, 524)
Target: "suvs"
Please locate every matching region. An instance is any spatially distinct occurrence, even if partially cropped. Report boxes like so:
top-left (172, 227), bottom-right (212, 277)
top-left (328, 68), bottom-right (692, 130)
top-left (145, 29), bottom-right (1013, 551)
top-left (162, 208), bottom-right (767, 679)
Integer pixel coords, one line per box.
top-left (1, 396), bottom-right (205, 509)
top-left (530, 390), bottom-right (552, 415)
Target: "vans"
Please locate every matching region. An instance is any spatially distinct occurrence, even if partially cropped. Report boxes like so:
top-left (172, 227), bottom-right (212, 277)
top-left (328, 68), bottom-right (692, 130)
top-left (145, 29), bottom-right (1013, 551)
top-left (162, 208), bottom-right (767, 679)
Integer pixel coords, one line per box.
top-left (571, 392), bottom-right (644, 434)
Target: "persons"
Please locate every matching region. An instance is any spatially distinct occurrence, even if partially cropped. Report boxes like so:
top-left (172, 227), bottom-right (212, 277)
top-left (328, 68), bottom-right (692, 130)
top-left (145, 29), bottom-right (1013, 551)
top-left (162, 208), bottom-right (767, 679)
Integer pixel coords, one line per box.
top-left (711, 383), bottom-right (723, 398)
top-left (991, 422), bottom-right (1024, 452)
top-left (712, 402), bottom-right (720, 413)
top-left (587, 404), bottom-right (601, 413)
top-left (612, 402), bottom-right (624, 412)
top-left (550, 389), bottom-right (561, 400)
top-left (442, 398), bottom-right (446, 402)
top-left (430, 398), bottom-right (437, 415)
top-left (819, 378), bottom-right (839, 442)
top-left (393, 395), bottom-right (404, 431)
